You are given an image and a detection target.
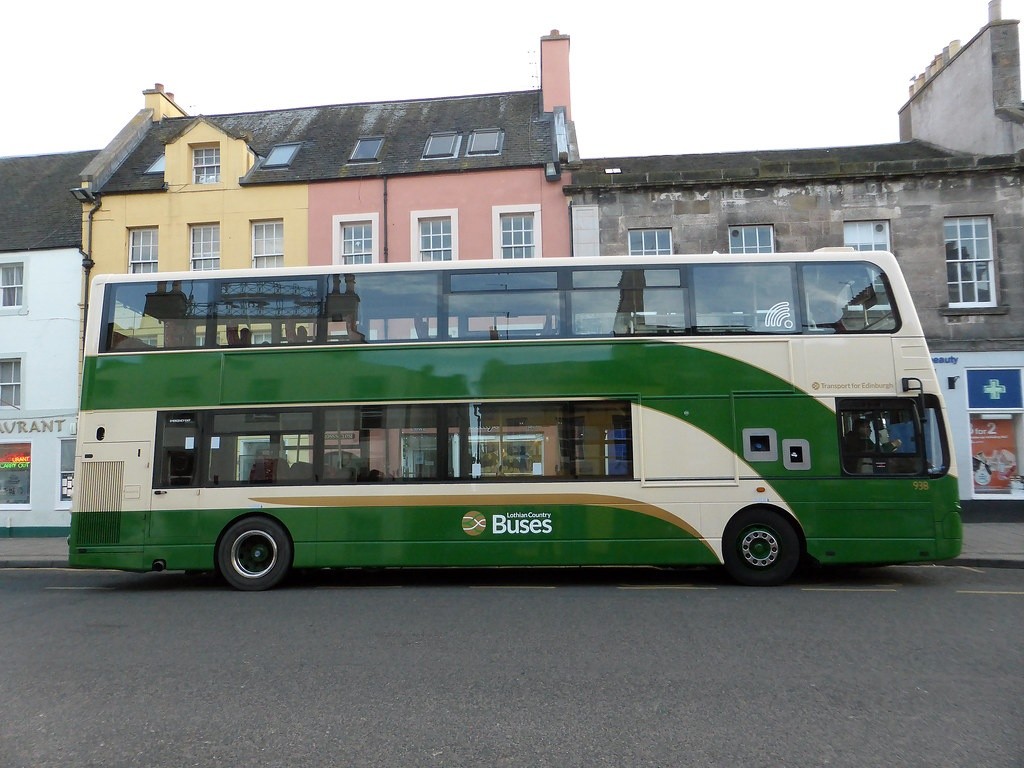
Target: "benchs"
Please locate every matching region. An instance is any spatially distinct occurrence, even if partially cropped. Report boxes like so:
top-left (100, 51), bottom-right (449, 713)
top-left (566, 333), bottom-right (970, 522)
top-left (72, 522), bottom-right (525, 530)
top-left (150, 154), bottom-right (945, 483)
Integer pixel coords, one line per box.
top-left (111, 330), bottom-right (158, 351)
top-left (167, 453), bottom-right (197, 487)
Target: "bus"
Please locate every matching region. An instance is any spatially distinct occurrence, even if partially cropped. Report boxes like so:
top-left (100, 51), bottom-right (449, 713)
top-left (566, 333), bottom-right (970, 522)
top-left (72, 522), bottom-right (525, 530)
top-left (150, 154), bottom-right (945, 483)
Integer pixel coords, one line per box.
top-left (66, 245), bottom-right (964, 593)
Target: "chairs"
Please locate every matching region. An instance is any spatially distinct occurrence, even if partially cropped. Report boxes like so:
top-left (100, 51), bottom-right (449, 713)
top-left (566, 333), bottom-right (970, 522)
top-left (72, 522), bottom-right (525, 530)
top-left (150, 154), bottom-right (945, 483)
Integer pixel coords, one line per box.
top-left (414, 316), bottom-right (429, 339)
top-left (239, 328), bottom-right (252, 344)
top-left (542, 313), bottom-right (553, 336)
top-left (296, 326), bottom-right (309, 345)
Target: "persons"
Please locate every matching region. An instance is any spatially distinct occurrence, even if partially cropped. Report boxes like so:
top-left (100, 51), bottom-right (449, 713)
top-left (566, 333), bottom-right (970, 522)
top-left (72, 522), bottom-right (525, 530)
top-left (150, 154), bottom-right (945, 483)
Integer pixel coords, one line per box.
top-left (844, 420), bottom-right (902, 473)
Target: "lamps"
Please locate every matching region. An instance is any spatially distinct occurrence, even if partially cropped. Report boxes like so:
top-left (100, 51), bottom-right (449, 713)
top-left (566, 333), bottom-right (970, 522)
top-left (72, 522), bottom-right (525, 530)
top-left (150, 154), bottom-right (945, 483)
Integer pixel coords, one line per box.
top-left (947, 376), bottom-right (960, 389)
top-left (69, 188), bottom-right (94, 203)
top-left (543, 160), bottom-right (562, 182)
